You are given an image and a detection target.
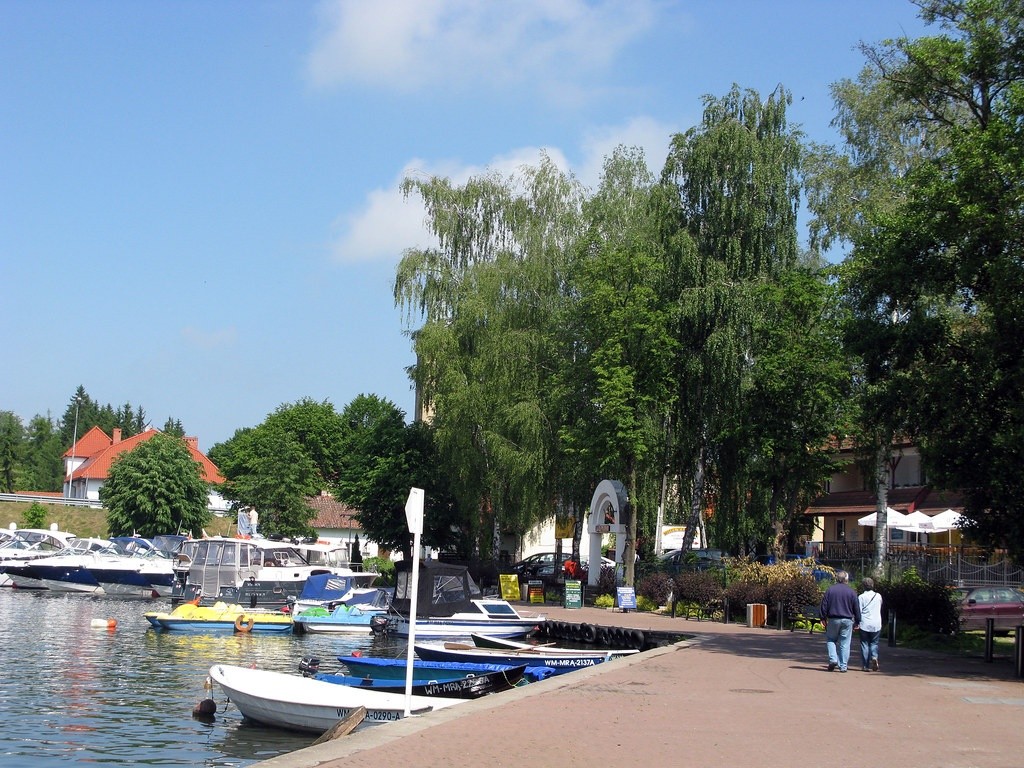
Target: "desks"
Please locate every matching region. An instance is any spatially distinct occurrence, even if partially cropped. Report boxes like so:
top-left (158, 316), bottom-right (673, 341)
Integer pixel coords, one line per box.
top-left (686, 606), bottom-right (719, 622)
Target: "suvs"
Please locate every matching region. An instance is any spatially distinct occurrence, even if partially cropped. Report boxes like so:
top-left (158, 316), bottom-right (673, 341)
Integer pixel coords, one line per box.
top-left (747, 553), bottom-right (849, 587)
top-left (632, 547), bottom-right (735, 584)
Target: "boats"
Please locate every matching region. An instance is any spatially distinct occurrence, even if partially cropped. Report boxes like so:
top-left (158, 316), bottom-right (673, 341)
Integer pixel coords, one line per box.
top-left (293, 605), bottom-right (387, 633)
top-left (0, 521), bottom-right (106, 564)
top-left (405, 641), bottom-right (613, 676)
top-left (151, 533), bottom-right (547, 626)
top-left (370, 615), bottom-right (548, 642)
top-left (0, 536), bottom-right (180, 600)
top-left (207, 663), bottom-right (475, 732)
top-left (335, 656), bottom-right (557, 684)
top-left (303, 661), bottom-right (530, 699)
top-left (470, 632), bottom-right (640, 662)
top-left (143, 598), bottom-right (295, 633)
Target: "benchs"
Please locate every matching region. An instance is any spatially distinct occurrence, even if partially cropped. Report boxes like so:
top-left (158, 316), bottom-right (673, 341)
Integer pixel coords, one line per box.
top-left (788, 605), bottom-right (821, 635)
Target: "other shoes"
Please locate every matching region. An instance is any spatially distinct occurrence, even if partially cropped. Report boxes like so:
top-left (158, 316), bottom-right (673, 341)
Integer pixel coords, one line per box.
top-left (828, 661), bottom-right (837, 671)
top-left (872, 658), bottom-right (879, 671)
top-left (863, 665), bottom-right (870, 672)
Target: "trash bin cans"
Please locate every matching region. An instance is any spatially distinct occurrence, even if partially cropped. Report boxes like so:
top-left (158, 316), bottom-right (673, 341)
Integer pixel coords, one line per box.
top-left (747, 603), bottom-right (767, 628)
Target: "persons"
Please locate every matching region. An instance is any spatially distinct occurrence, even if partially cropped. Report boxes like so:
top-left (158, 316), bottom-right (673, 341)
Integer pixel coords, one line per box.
top-left (247, 505), bottom-right (259, 533)
top-left (856, 577), bottom-right (883, 672)
top-left (426, 554), bottom-right (433, 562)
top-left (821, 570), bottom-right (861, 673)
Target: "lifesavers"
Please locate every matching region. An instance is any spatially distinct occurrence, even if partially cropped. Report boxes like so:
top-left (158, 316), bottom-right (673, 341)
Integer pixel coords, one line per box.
top-left (537, 622), bottom-right (649, 651)
top-left (235, 534), bottom-right (251, 540)
top-left (234, 614), bottom-right (254, 632)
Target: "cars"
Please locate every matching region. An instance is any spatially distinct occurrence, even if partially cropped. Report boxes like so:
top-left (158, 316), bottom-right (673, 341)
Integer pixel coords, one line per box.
top-left (930, 584), bottom-right (1024, 635)
top-left (509, 552), bottom-right (617, 580)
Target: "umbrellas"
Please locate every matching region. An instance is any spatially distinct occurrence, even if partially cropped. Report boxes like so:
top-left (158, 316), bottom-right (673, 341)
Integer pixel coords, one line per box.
top-left (896, 510), bottom-right (934, 547)
top-left (927, 508), bottom-right (977, 565)
top-left (858, 505), bottom-right (911, 555)
